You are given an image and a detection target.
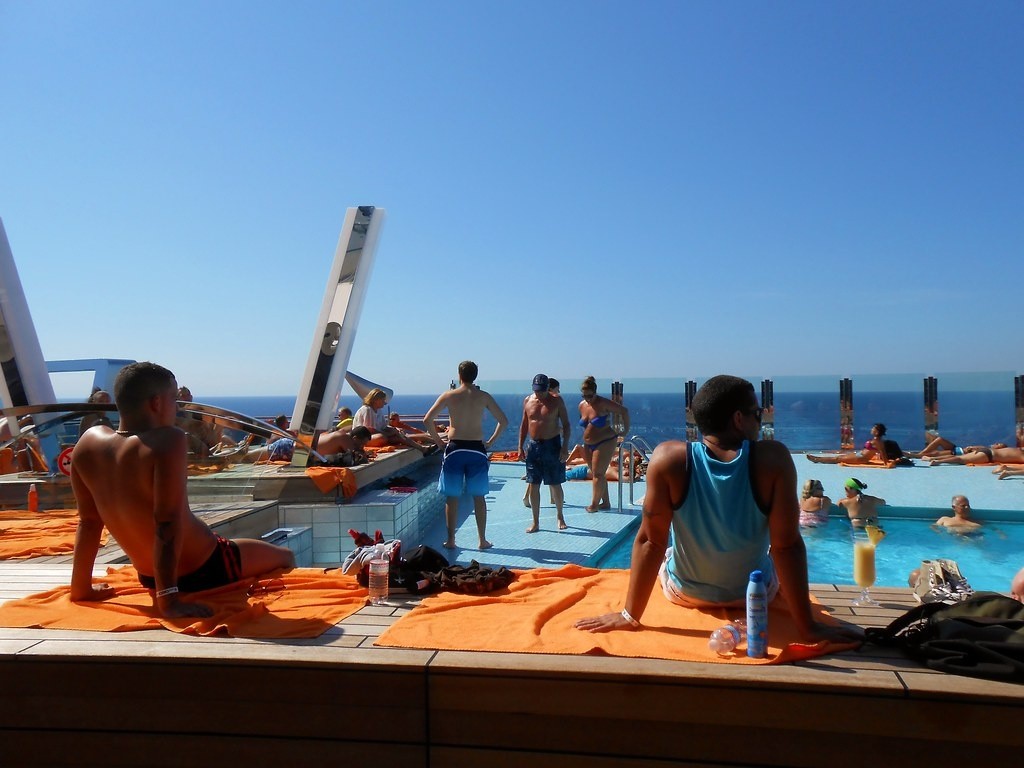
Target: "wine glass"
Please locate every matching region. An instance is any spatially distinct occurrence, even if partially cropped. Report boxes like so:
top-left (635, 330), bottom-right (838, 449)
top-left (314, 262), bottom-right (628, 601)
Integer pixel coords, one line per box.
top-left (851, 532), bottom-right (880, 607)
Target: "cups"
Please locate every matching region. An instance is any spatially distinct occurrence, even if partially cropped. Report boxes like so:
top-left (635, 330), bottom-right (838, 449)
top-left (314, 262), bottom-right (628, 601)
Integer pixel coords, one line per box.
top-left (876, 453), bottom-right (880, 459)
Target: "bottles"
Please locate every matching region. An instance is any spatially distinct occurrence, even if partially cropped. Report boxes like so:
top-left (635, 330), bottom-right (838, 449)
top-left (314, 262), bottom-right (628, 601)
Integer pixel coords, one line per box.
top-left (368, 543), bottom-right (390, 607)
top-left (28, 484), bottom-right (38, 512)
top-left (746, 570), bottom-right (768, 659)
top-left (707, 616), bottom-right (748, 654)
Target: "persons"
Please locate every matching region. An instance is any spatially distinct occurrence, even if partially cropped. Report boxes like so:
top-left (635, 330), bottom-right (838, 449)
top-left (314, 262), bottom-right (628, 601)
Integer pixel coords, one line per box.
top-left (578, 376), bottom-right (630, 512)
top-left (70, 362), bottom-right (295, 618)
top-left (799, 479), bottom-right (832, 524)
top-left (908, 436), bottom-right (1024, 466)
top-left (233, 426), bottom-right (371, 463)
top-left (353, 387), bottom-right (438, 457)
top-left (517, 373), bottom-right (571, 534)
top-left (390, 411), bottom-right (400, 427)
top-left (79, 391), bottom-right (114, 438)
top-left (992, 464), bottom-right (1024, 480)
top-left (423, 361), bottom-right (508, 548)
top-left (336, 407), bottom-right (353, 428)
top-left (835, 477), bottom-right (886, 525)
top-left (807, 423), bottom-right (889, 465)
top-left (178, 387), bottom-right (193, 402)
top-left (276, 415), bottom-right (289, 430)
top-left (937, 495), bottom-right (981, 526)
top-left (576, 375), bottom-right (861, 644)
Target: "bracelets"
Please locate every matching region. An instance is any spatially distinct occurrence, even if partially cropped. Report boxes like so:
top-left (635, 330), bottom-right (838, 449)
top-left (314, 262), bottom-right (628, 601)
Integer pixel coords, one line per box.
top-left (156, 587), bottom-right (178, 598)
top-left (622, 609), bottom-right (640, 627)
